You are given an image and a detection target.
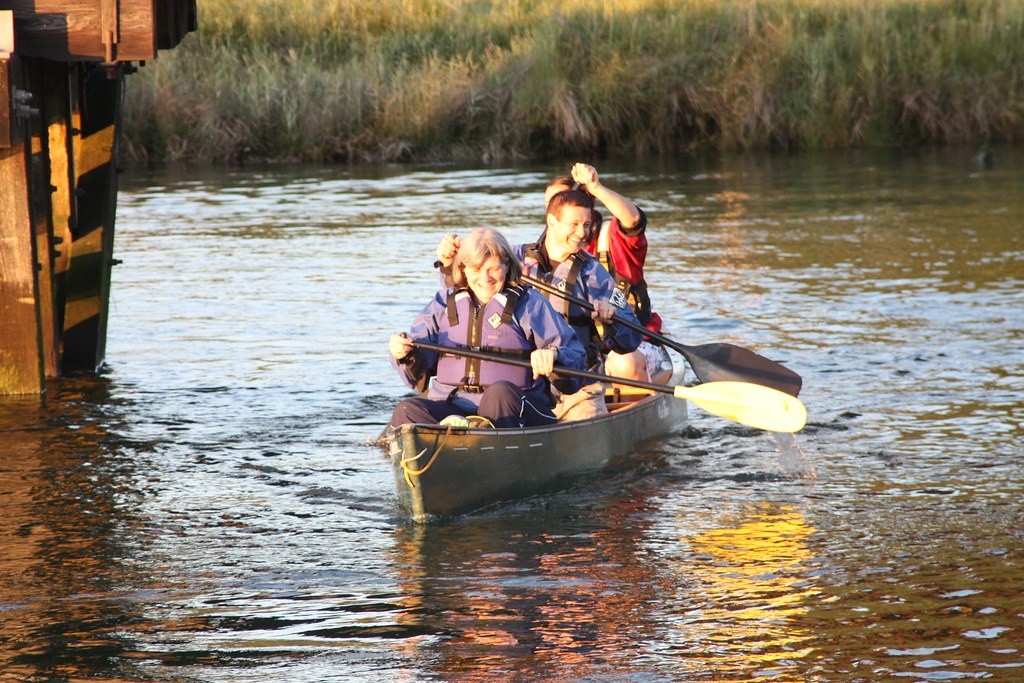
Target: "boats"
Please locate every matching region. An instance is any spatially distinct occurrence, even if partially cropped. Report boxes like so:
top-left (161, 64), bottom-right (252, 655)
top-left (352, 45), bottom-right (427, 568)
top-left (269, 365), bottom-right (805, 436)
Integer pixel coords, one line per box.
top-left (387, 326), bottom-right (688, 521)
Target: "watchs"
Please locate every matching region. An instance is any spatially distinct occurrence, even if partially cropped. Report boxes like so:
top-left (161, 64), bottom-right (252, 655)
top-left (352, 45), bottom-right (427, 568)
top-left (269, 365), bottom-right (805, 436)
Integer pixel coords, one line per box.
top-left (543, 343), bottom-right (558, 361)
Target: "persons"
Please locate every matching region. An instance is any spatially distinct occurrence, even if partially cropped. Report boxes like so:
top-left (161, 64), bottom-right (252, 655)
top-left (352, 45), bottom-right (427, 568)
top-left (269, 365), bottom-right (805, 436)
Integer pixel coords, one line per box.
top-left (536, 163), bottom-right (673, 397)
top-left (436, 188), bottom-right (644, 424)
top-left (382, 226), bottom-right (589, 466)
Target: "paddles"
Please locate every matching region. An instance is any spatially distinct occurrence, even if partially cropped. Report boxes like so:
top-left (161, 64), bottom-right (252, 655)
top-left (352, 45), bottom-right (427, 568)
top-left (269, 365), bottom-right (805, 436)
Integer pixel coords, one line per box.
top-left (455, 246), bottom-right (802, 398)
top-left (400, 332), bottom-right (807, 433)
